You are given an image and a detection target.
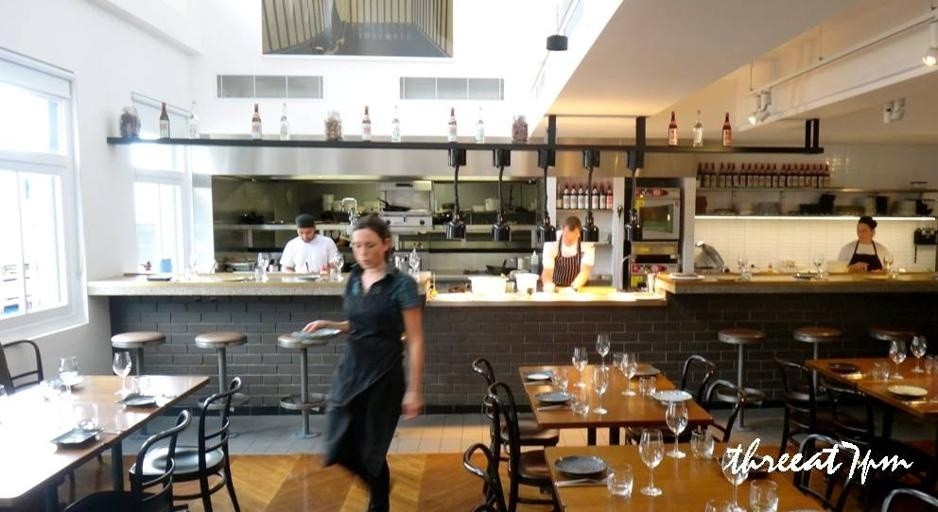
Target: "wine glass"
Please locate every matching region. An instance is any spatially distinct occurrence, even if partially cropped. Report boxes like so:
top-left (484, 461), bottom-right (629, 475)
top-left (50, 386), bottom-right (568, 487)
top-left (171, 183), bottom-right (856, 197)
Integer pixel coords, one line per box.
top-left (570, 334), bottom-right (637, 418)
top-left (257, 253), bottom-right (270, 282)
top-left (910, 334), bottom-right (927, 374)
top-left (637, 429), bottom-right (664, 497)
top-left (721, 448), bottom-right (749, 512)
top-left (111, 351), bottom-right (134, 396)
top-left (888, 340), bottom-right (908, 380)
top-left (664, 400), bottom-right (689, 458)
top-left (332, 253), bottom-right (344, 282)
top-left (59, 357), bottom-right (79, 399)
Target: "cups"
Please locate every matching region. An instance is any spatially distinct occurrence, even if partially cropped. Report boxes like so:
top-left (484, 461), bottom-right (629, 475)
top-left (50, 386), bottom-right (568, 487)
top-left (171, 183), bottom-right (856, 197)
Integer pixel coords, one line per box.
top-left (607, 462), bottom-right (634, 497)
top-left (394, 248), bottom-right (420, 277)
top-left (872, 360), bottom-right (889, 383)
top-left (518, 257), bottom-right (530, 271)
top-left (736, 251), bottom-right (896, 282)
top-left (76, 402), bottom-right (99, 431)
top-left (549, 368), bottom-right (569, 391)
top-left (690, 430), bottom-right (713, 458)
top-left (255, 267), bottom-right (265, 283)
top-left (638, 377), bottom-right (656, 398)
top-left (924, 355), bottom-right (937, 375)
top-left (704, 480), bottom-right (779, 512)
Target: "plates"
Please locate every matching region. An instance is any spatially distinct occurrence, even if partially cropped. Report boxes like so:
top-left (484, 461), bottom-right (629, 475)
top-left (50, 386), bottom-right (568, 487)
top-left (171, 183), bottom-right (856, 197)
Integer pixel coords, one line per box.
top-left (296, 275), bottom-right (322, 281)
top-left (825, 362), bottom-right (859, 371)
top-left (555, 455), bottom-right (608, 477)
top-left (532, 390), bottom-right (571, 404)
top-left (218, 277), bottom-right (253, 282)
top-left (650, 389), bottom-right (693, 406)
top-left (717, 453), bottom-right (774, 475)
top-left (53, 427), bottom-right (105, 449)
top-left (888, 384), bottom-right (929, 397)
top-left (53, 374), bottom-right (83, 388)
top-left (120, 393), bottom-right (158, 409)
top-left (523, 370), bottom-right (554, 380)
top-left (146, 277), bottom-right (173, 282)
top-left (630, 366), bottom-right (662, 377)
top-left (733, 196), bottom-right (918, 215)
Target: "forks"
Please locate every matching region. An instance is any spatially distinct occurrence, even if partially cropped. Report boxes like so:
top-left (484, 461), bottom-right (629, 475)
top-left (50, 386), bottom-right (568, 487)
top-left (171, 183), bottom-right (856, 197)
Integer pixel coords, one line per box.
top-left (554, 472), bottom-right (615, 486)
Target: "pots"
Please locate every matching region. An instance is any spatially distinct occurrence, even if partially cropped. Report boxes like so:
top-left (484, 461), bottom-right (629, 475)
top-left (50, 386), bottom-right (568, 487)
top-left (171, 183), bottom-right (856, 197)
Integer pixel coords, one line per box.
top-left (376, 198), bottom-right (411, 211)
top-left (486, 260), bottom-right (514, 275)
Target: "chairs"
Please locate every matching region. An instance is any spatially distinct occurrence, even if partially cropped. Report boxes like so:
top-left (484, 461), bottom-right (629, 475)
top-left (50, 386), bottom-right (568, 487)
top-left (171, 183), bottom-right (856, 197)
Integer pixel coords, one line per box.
top-left (0, 323), bottom-right (936, 512)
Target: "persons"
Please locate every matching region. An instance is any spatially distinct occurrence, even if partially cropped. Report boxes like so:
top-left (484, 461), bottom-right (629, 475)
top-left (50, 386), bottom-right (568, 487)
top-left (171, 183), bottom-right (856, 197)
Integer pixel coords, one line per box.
top-left (540, 216), bottom-right (596, 292)
top-left (277, 214), bottom-right (341, 272)
top-left (304, 213), bottom-right (425, 512)
top-left (834, 216), bottom-right (894, 272)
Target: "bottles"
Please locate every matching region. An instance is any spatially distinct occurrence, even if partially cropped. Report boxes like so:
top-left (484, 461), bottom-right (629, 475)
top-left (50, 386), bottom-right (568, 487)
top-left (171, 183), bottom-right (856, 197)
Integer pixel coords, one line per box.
top-left (529, 250), bottom-right (539, 273)
top-left (694, 163), bottom-right (832, 188)
top-left (556, 182), bottom-right (614, 210)
top-left (721, 112), bottom-right (733, 150)
top-left (119, 101), bottom-right (487, 146)
top-left (693, 108), bottom-right (704, 148)
top-left (668, 110), bottom-right (678, 146)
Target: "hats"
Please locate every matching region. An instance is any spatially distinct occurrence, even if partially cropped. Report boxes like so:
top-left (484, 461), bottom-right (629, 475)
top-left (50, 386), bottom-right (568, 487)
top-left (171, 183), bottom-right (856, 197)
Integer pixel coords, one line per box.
top-left (858, 214), bottom-right (879, 230)
top-left (295, 213), bottom-right (315, 227)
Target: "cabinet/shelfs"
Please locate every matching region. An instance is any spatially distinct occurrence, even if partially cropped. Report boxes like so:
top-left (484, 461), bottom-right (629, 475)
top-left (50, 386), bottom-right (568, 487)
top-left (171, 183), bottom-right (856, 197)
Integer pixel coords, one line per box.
top-left (556, 207), bottom-right (612, 247)
top-left (694, 186), bottom-right (936, 223)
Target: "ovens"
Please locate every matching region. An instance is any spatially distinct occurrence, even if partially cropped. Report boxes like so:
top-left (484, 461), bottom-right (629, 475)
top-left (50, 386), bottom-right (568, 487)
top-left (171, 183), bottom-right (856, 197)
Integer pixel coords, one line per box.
top-left (625, 183), bottom-right (681, 291)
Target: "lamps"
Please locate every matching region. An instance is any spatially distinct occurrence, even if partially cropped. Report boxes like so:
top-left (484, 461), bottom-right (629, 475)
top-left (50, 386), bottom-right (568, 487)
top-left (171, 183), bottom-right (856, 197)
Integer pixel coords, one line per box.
top-left (882, 96), bottom-right (906, 125)
top-left (546, 2), bottom-right (568, 53)
top-left (579, 147), bottom-right (599, 243)
top-left (534, 146), bottom-right (557, 245)
top-left (491, 148), bottom-right (512, 243)
top-left (445, 146), bottom-right (467, 241)
top-left (625, 149), bottom-right (644, 244)
top-left (746, 89), bottom-right (773, 126)
